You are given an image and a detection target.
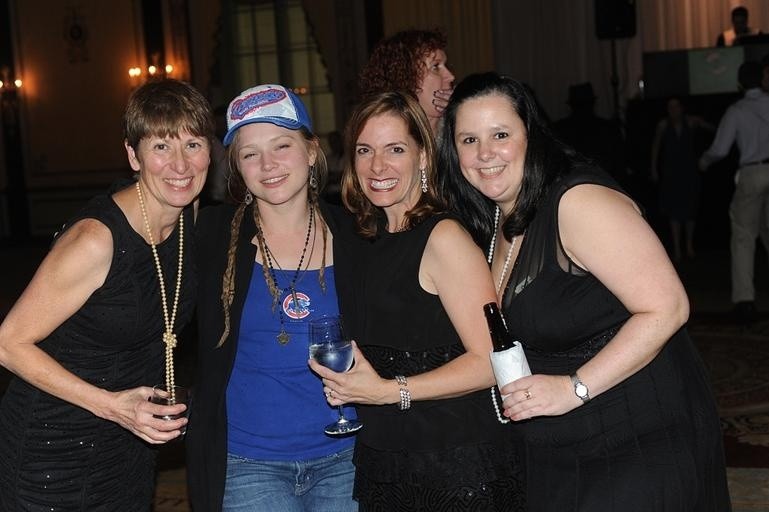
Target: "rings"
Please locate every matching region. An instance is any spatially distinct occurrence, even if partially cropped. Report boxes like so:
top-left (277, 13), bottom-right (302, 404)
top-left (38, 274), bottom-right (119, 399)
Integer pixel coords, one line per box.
top-left (329, 390), bottom-right (334, 398)
top-left (525, 391), bottom-right (532, 400)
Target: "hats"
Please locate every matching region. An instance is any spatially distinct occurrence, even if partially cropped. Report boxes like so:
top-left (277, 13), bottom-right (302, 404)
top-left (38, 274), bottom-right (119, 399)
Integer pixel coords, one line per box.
top-left (223, 84), bottom-right (314, 147)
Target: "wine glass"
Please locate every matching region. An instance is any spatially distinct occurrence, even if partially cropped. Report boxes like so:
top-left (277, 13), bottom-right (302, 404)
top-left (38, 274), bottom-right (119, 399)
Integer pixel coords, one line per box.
top-left (308, 312), bottom-right (366, 437)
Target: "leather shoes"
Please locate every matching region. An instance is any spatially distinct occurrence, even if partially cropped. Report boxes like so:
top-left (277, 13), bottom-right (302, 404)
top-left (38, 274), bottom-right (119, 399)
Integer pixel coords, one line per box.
top-left (728, 301), bottom-right (757, 323)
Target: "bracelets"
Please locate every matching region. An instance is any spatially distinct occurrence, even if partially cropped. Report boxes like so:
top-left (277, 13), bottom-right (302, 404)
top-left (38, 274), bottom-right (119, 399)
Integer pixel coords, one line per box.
top-left (395, 375), bottom-right (411, 411)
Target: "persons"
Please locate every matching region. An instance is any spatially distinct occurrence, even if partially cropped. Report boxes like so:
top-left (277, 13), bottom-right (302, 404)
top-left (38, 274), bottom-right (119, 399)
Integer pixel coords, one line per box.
top-left (308, 90), bottom-right (528, 511)
top-left (184, 82), bottom-right (359, 512)
top-left (430, 70), bottom-right (732, 512)
top-left (359, 26), bottom-right (456, 138)
top-left (552, 6), bottom-right (768, 328)
top-left (0, 77), bottom-right (216, 512)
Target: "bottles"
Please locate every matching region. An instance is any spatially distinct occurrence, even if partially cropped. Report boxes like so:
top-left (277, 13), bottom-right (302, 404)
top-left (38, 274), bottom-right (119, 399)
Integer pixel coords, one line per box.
top-left (481, 301), bottom-right (535, 421)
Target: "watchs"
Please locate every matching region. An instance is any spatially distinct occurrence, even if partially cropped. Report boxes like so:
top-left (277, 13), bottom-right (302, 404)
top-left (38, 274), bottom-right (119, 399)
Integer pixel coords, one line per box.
top-left (570, 372), bottom-right (591, 404)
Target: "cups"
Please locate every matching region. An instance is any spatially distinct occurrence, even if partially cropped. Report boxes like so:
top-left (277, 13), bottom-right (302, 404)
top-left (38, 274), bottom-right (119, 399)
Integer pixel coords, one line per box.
top-left (148, 384), bottom-right (193, 443)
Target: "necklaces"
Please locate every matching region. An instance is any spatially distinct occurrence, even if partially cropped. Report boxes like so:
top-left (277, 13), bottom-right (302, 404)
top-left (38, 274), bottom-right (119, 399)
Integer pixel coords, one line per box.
top-left (135, 181), bottom-right (184, 403)
top-left (488, 203), bottom-right (517, 425)
top-left (255, 200), bottom-right (317, 346)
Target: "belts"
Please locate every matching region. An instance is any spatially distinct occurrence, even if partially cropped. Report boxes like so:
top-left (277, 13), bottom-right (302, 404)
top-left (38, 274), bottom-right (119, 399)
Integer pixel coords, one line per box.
top-left (740, 157), bottom-right (769, 166)
